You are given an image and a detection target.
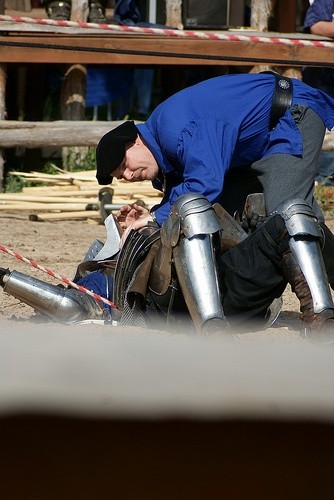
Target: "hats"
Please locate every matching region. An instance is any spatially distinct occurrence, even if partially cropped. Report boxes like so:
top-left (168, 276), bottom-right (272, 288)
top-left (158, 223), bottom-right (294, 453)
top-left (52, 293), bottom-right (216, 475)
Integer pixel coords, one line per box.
top-left (95, 118), bottom-right (138, 186)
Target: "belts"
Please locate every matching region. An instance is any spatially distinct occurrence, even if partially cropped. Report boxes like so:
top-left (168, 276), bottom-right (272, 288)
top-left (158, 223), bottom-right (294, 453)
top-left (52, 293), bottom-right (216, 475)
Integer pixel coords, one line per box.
top-left (262, 69), bottom-right (292, 131)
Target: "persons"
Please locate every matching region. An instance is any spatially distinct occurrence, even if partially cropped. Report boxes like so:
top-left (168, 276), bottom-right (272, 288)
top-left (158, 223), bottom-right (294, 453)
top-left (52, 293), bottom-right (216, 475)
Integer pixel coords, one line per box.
top-left (96, 71), bottom-right (334, 293)
top-left (0, 192), bottom-right (334, 337)
top-left (85, 0), bottom-right (155, 120)
top-left (301, 0), bottom-right (334, 99)
